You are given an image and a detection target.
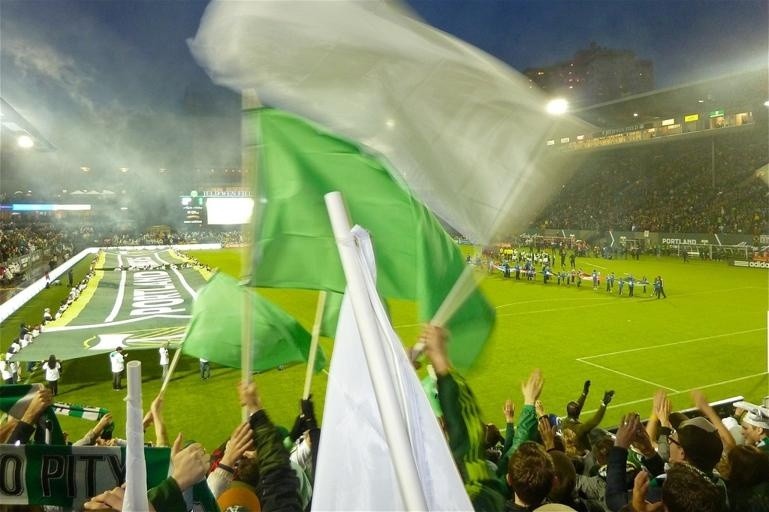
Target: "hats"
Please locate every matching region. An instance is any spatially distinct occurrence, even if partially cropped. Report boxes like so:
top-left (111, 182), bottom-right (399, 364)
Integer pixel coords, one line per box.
top-left (670, 400), bottom-right (768, 434)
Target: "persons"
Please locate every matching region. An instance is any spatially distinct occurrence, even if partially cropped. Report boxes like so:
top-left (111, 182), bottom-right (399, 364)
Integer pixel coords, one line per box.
top-left (455, 234), bottom-right (667, 301)
top-left (0, 211), bottom-right (247, 321)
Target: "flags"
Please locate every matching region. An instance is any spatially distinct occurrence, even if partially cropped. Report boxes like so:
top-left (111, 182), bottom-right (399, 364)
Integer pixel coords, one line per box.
top-left (185, 2), bottom-right (600, 247)
top-left (244, 106), bottom-right (503, 385)
top-left (305, 223), bottom-right (474, 512)
top-left (183, 269), bottom-right (323, 379)
top-left (317, 290), bottom-right (389, 332)
